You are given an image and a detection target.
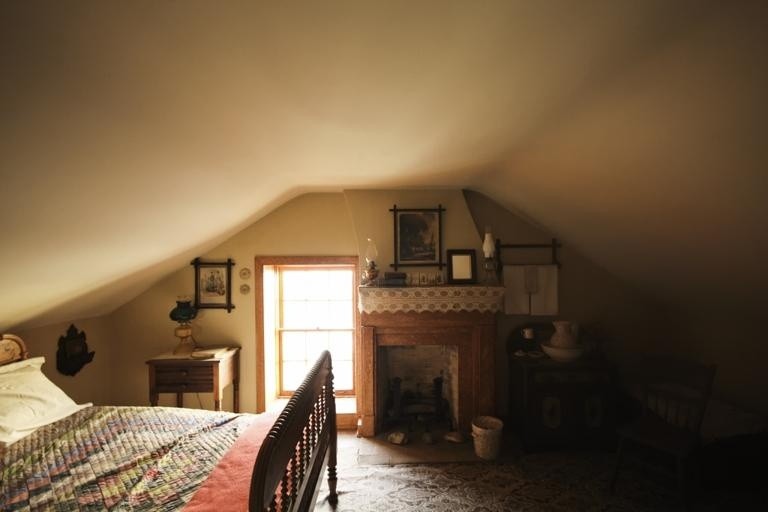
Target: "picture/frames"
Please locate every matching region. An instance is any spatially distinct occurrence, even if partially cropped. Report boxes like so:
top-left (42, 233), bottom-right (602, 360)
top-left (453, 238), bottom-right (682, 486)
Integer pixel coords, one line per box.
top-left (189, 256), bottom-right (236, 314)
top-left (390, 203), bottom-right (447, 271)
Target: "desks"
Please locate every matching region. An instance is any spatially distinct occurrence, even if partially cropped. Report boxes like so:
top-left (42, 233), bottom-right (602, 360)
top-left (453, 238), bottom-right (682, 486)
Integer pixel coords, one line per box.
top-left (144, 347), bottom-right (242, 413)
top-left (356, 284), bottom-right (503, 439)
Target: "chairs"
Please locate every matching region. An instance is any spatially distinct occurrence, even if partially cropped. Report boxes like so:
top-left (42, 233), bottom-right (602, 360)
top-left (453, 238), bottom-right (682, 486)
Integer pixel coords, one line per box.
top-left (609, 357), bottom-right (717, 495)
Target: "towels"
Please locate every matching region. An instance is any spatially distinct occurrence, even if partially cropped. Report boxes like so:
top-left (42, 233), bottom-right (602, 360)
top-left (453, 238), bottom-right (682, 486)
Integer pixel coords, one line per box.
top-left (502, 264), bottom-right (559, 318)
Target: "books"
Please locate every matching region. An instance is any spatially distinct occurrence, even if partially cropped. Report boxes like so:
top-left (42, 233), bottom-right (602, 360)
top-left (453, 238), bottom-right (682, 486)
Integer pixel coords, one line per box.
top-left (191, 344), bottom-right (231, 359)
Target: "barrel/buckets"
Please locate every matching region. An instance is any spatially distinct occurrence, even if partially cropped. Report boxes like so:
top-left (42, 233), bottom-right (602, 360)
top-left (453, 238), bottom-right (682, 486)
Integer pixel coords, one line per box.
top-left (470, 416), bottom-right (503, 457)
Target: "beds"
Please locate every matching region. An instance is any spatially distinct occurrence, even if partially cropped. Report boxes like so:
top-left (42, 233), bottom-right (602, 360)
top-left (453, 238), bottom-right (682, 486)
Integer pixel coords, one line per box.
top-left (1, 333), bottom-right (339, 511)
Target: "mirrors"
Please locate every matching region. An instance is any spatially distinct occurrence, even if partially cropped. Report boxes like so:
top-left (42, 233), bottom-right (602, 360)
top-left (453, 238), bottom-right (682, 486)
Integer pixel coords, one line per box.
top-left (446, 248), bottom-right (477, 284)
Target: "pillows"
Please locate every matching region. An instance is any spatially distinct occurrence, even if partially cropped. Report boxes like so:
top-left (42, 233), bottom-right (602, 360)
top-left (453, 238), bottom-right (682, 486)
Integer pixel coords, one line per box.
top-left (0, 356), bottom-right (94, 449)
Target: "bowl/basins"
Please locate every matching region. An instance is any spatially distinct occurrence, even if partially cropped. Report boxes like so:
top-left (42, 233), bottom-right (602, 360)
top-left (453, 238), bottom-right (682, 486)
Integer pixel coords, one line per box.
top-left (541, 341), bottom-right (586, 363)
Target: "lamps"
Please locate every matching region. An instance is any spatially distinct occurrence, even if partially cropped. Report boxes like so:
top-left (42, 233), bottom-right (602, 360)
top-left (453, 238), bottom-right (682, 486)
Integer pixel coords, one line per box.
top-left (364, 233), bottom-right (380, 287)
top-left (479, 232), bottom-right (499, 287)
